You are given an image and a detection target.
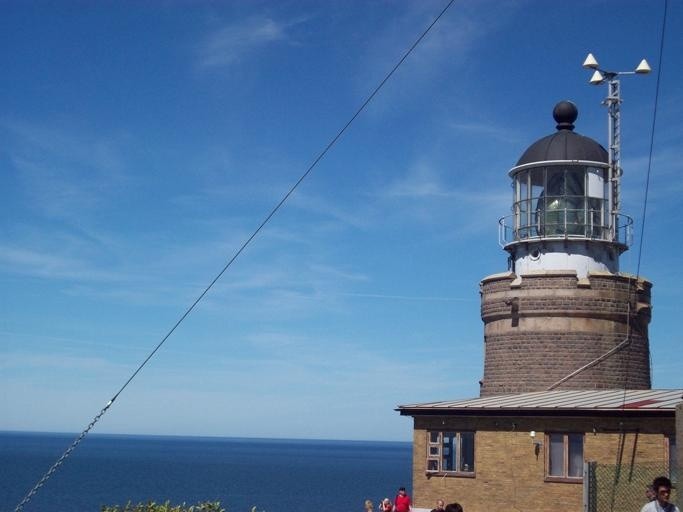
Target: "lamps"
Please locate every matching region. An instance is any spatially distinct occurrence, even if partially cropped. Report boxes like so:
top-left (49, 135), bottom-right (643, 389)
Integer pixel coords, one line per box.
top-left (529, 430), bottom-right (540, 445)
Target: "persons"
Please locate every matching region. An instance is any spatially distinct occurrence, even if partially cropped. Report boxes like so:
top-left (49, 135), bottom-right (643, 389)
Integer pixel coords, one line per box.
top-left (644, 483), bottom-right (657, 502)
top-left (391, 486), bottom-right (412, 512)
top-left (378, 497), bottom-right (393, 512)
top-left (363, 499), bottom-right (375, 512)
top-left (641, 476), bottom-right (680, 512)
top-left (430, 498), bottom-right (445, 512)
